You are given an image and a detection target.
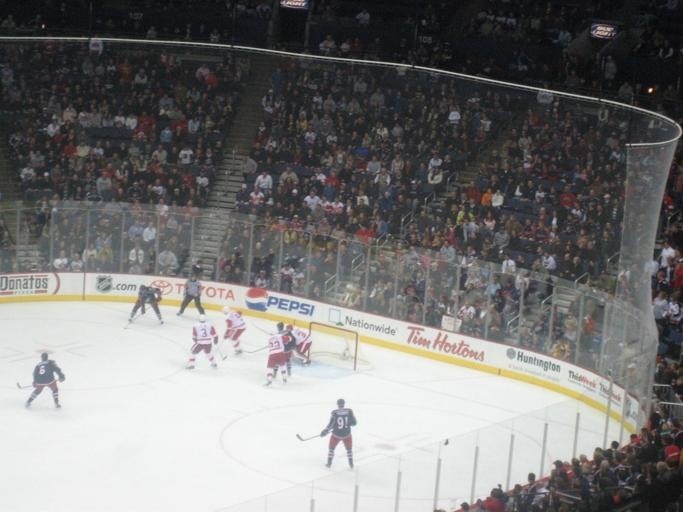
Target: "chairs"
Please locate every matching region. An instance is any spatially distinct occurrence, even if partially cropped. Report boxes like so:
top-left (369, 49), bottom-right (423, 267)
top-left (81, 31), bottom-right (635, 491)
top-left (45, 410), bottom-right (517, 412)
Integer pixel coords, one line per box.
top-left (0, 32), bottom-right (683, 377)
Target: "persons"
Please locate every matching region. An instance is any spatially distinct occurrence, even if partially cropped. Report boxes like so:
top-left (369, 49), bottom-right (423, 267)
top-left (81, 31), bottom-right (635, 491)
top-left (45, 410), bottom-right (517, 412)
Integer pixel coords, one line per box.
top-left (176, 273), bottom-right (204, 317)
top-left (223, 306), bottom-right (247, 354)
top-left (321, 399), bottom-right (357, 468)
top-left (218, 1), bottom-right (682, 340)
top-left (462, 331), bottom-right (681, 511)
top-left (129, 285), bottom-right (163, 324)
top-left (2, 1), bottom-right (274, 276)
top-left (186, 315), bottom-right (219, 370)
top-left (263, 322), bottom-right (312, 386)
top-left (25, 353), bottom-right (65, 407)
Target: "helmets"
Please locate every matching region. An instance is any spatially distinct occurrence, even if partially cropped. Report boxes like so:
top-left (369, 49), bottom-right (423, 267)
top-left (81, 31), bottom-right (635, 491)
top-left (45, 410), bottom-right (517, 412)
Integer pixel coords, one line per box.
top-left (337, 399), bottom-right (345, 406)
top-left (41, 353), bottom-right (47, 361)
top-left (199, 314), bottom-right (205, 321)
top-left (276, 322), bottom-right (293, 330)
top-left (224, 306), bottom-right (230, 313)
top-left (140, 285), bottom-right (146, 291)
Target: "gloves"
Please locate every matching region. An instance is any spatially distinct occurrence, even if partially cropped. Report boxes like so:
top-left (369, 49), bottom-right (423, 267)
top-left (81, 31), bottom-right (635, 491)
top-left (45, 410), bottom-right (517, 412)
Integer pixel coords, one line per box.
top-left (213, 336), bottom-right (218, 344)
top-left (141, 308), bottom-right (146, 314)
top-left (157, 297), bottom-right (162, 302)
top-left (58, 375), bottom-right (65, 381)
top-left (321, 429), bottom-right (328, 436)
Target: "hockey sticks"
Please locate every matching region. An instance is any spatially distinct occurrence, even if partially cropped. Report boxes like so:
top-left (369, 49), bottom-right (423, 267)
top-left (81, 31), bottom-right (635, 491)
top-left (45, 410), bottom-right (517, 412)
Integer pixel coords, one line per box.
top-left (296, 430), bottom-right (332, 441)
top-left (244, 345), bottom-right (270, 353)
top-left (17, 383), bottom-right (32, 389)
